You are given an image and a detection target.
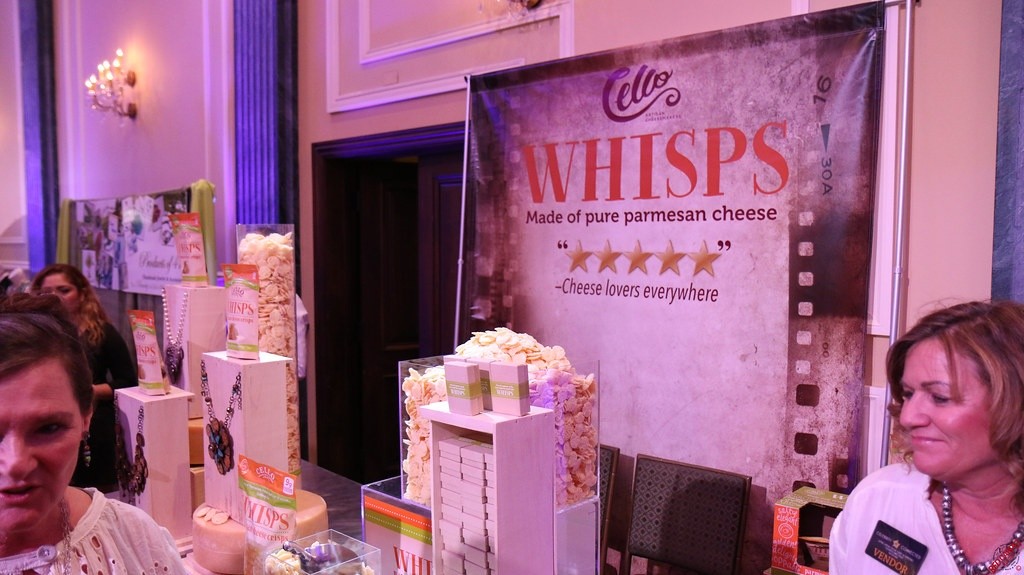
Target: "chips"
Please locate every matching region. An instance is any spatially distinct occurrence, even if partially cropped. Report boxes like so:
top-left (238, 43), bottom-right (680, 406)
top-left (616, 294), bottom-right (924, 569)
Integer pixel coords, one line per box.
top-left (238, 232), bottom-right (301, 476)
top-left (264, 539), bottom-right (374, 575)
top-left (196, 506), bottom-right (228, 524)
top-left (402, 326), bottom-right (599, 511)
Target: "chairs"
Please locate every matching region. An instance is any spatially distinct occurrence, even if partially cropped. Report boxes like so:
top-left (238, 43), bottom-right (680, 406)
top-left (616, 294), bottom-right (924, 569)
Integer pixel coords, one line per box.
top-left (620, 455), bottom-right (752, 575)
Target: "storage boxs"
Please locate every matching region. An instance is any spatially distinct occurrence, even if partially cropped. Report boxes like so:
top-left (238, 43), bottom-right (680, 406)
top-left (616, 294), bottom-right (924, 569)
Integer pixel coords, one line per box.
top-left (771, 486), bottom-right (850, 575)
top-left (438, 351), bottom-right (531, 575)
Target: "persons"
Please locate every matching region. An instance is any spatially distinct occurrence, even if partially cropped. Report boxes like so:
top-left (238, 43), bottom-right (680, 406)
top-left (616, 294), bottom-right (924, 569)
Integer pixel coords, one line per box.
top-left (76, 203), bottom-right (160, 287)
top-left (0, 264), bottom-right (187, 575)
top-left (828, 300), bottom-right (1024, 575)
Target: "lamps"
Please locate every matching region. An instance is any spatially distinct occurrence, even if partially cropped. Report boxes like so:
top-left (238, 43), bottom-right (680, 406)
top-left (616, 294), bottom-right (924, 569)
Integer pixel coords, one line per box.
top-left (85, 48), bottom-right (137, 120)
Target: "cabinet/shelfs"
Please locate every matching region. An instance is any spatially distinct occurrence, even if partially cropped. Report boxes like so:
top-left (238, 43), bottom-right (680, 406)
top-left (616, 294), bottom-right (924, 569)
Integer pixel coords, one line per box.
top-left (420, 400), bottom-right (600, 575)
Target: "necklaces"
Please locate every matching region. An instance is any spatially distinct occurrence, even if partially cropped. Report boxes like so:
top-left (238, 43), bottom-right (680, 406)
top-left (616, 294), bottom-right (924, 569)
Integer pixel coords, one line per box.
top-left (60, 497), bottom-right (71, 575)
top-left (941, 485), bottom-right (1024, 575)
